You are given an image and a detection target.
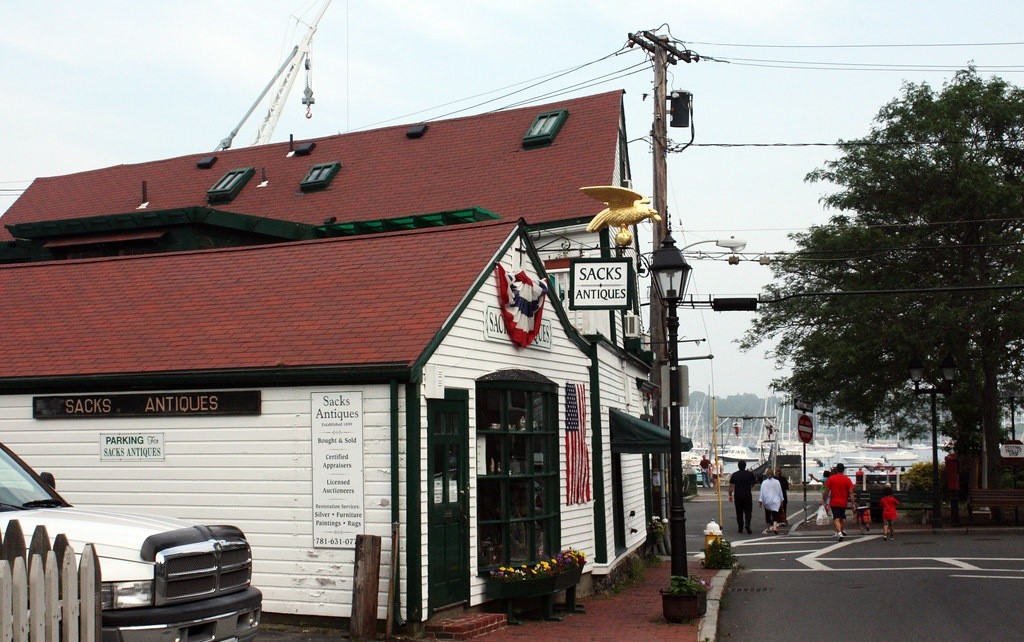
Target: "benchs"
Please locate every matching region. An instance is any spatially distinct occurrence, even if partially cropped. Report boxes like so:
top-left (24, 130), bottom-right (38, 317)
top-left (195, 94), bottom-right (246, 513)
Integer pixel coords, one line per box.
top-left (846, 489), bottom-right (939, 528)
top-left (967, 488), bottom-right (1024, 527)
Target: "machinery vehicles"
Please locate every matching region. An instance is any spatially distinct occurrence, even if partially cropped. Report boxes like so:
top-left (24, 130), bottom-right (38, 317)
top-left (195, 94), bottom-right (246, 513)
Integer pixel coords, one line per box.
top-left (217, 0), bottom-right (332, 152)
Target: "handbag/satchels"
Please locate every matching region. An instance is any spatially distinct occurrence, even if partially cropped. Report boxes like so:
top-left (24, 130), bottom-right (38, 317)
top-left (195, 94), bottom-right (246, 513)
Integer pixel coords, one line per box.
top-left (816, 504), bottom-right (830, 526)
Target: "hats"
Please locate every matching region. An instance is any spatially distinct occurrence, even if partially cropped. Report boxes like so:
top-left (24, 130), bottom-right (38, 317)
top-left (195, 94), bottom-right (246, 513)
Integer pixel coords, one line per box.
top-left (833, 463), bottom-right (847, 470)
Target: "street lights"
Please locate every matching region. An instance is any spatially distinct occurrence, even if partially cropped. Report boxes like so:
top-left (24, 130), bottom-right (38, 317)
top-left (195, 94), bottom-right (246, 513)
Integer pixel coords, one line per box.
top-left (648, 236), bottom-right (694, 590)
top-left (910, 352), bottom-right (956, 529)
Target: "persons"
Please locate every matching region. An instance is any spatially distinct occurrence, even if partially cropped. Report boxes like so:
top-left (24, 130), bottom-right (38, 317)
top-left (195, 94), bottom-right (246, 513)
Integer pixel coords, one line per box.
top-left (758, 470), bottom-right (789, 533)
top-left (728, 460), bottom-right (757, 534)
top-left (890, 466), bottom-right (897, 473)
top-left (809, 470), bottom-right (831, 506)
top-left (901, 467), bottom-right (905, 472)
top-left (700, 455), bottom-right (712, 488)
top-left (856, 467), bottom-right (864, 484)
top-left (878, 468), bottom-right (885, 473)
top-left (823, 463), bottom-right (856, 540)
top-left (711, 457), bottom-right (724, 494)
top-left (878, 488), bottom-right (900, 541)
top-left (868, 466), bottom-right (875, 473)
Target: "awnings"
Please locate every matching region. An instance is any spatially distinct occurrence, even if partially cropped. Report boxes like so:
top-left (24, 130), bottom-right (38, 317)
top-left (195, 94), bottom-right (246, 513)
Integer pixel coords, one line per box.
top-left (610, 405), bottom-right (693, 453)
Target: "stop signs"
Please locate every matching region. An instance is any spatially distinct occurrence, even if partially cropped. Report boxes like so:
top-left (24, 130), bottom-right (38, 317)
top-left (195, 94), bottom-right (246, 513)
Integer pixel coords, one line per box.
top-left (798, 413), bottom-right (815, 444)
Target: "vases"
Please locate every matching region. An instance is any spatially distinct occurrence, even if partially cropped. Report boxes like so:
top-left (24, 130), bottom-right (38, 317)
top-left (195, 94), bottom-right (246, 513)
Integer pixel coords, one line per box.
top-left (486, 566), bottom-right (586, 625)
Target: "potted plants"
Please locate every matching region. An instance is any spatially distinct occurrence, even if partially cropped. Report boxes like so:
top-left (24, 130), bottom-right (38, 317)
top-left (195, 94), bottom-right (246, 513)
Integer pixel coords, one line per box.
top-left (659, 575), bottom-right (711, 623)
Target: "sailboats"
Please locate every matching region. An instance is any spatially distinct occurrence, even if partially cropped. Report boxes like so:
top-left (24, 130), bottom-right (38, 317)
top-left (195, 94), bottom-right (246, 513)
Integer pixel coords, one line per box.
top-left (681, 385), bottom-right (949, 475)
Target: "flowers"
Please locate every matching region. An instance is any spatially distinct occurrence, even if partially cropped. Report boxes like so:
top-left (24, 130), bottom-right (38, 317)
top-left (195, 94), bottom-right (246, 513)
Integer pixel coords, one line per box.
top-left (489, 546), bottom-right (587, 584)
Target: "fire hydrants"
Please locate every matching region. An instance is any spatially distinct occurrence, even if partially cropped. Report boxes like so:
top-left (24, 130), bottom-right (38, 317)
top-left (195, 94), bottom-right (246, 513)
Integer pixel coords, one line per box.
top-left (702, 518), bottom-right (724, 566)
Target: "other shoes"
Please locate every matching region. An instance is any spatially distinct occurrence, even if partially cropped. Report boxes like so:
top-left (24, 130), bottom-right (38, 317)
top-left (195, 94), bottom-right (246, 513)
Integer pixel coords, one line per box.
top-left (837, 532), bottom-right (844, 543)
top-left (858, 523), bottom-right (862, 528)
top-left (773, 528), bottom-right (778, 534)
top-left (738, 528), bottom-right (743, 533)
top-left (842, 530), bottom-right (847, 536)
top-left (766, 529), bottom-right (771, 533)
top-left (745, 526), bottom-right (752, 532)
top-left (884, 536), bottom-right (888, 541)
top-left (890, 535), bottom-right (895, 538)
top-left (865, 524), bottom-right (870, 531)
top-left (832, 532), bottom-right (838, 538)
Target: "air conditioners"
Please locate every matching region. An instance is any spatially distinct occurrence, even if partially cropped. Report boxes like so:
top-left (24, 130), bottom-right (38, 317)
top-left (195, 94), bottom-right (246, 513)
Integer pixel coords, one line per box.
top-left (625, 314), bottom-right (643, 337)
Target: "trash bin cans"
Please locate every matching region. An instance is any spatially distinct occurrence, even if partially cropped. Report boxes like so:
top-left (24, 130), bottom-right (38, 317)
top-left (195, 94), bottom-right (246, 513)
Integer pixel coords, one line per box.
top-left (684, 474), bottom-right (697, 495)
top-left (946, 454), bottom-right (979, 493)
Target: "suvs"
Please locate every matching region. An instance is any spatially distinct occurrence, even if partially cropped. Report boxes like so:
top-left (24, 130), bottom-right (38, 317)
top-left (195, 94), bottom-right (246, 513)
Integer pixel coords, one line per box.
top-left (0, 442), bottom-right (264, 642)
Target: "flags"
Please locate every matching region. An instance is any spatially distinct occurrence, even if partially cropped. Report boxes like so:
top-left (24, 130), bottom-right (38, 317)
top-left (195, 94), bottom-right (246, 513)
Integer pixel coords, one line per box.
top-left (565, 384), bottom-right (591, 508)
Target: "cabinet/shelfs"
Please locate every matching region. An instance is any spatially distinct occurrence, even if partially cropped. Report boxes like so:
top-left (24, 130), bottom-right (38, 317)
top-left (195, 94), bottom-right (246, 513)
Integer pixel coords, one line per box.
top-left (476, 380), bottom-right (558, 577)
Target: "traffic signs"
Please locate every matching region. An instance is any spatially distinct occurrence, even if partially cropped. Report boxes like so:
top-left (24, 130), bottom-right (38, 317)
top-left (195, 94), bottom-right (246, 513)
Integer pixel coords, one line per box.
top-left (793, 397), bottom-right (813, 413)
